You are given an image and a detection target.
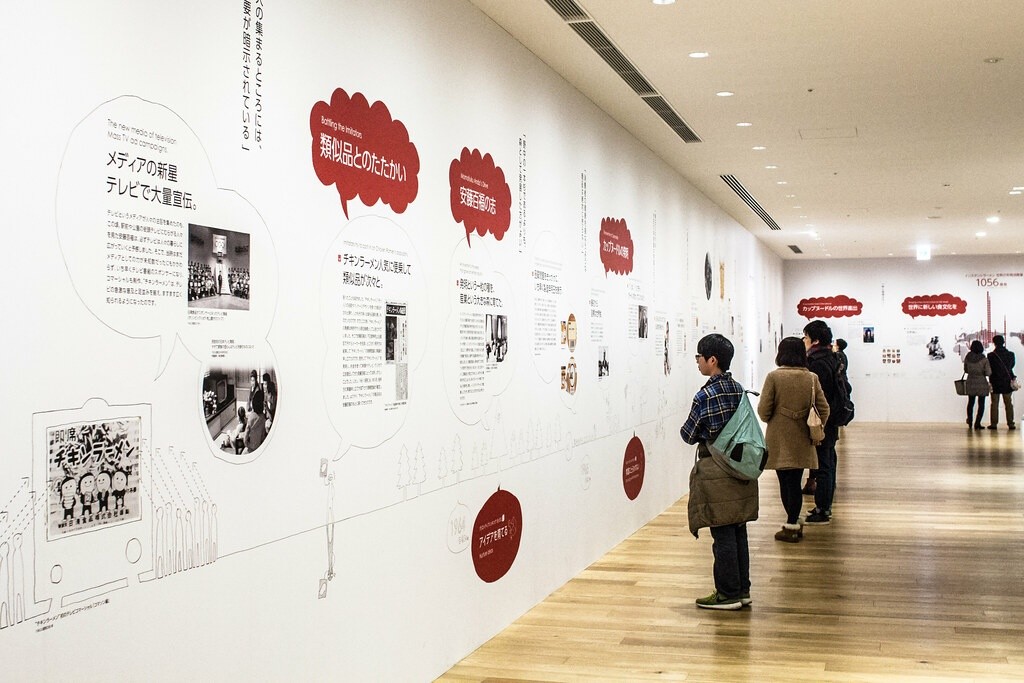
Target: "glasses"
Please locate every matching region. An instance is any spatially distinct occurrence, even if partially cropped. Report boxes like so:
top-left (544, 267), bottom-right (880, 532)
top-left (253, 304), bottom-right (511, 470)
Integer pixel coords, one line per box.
top-left (695, 354), bottom-right (703, 360)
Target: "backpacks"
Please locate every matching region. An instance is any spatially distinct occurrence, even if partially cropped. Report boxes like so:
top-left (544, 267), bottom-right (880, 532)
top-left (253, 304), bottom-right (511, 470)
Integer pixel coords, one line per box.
top-left (811, 355), bottom-right (854, 427)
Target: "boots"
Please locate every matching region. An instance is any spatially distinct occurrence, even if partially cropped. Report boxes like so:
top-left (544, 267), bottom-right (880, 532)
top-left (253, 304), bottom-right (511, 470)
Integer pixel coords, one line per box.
top-left (802, 478), bottom-right (816, 495)
top-left (775, 518), bottom-right (804, 543)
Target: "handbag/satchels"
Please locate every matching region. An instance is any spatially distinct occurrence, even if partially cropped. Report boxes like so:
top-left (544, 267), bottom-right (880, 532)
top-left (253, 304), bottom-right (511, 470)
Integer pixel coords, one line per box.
top-left (705, 383), bottom-right (768, 480)
top-left (807, 372), bottom-right (825, 442)
top-left (954, 372), bottom-right (968, 395)
top-left (1010, 378), bottom-right (1021, 391)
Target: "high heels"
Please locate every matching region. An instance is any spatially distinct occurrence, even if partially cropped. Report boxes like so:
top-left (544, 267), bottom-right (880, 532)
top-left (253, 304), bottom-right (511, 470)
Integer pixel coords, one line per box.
top-left (966, 418), bottom-right (973, 428)
top-left (974, 422), bottom-right (985, 430)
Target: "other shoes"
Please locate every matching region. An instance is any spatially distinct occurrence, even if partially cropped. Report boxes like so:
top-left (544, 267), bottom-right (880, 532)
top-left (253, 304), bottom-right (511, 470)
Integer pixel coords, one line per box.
top-left (987, 425), bottom-right (997, 429)
top-left (1009, 425), bottom-right (1016, 430)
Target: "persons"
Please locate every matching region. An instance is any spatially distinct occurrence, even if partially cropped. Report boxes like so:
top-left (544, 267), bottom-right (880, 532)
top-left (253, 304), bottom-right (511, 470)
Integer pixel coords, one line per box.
top-left (226, 369), bottom-right (277, 456)
top-left (801, 320), bottom-right (852, 525)
top-left (681, 334), bottom-right (759, 610)
top-left (987, 335), bottom-right (1016, 430)
top-left (963, 340), bottom-right (992, 430)
top-left (927, 336), bottom-right (942, 355)
top-left (757, 336), bottom-right (830, 543)
top-left (188, 261), bottom-right (250, 303)
top-left (602, 351), bottom-right (608, 376)
top-left (487, 337), bottom-right (507, 362)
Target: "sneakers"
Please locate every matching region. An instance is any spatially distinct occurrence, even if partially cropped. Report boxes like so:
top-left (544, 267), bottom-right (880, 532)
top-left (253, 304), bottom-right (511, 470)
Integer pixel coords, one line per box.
top-left (803, 513), bottom-right (830, 526)
top-left (696, 591), bottom-right (742, 610)
top-left (740, 591), bottom-right (752, 604)
top-left (806, 507), bottom-right (832, 521)
